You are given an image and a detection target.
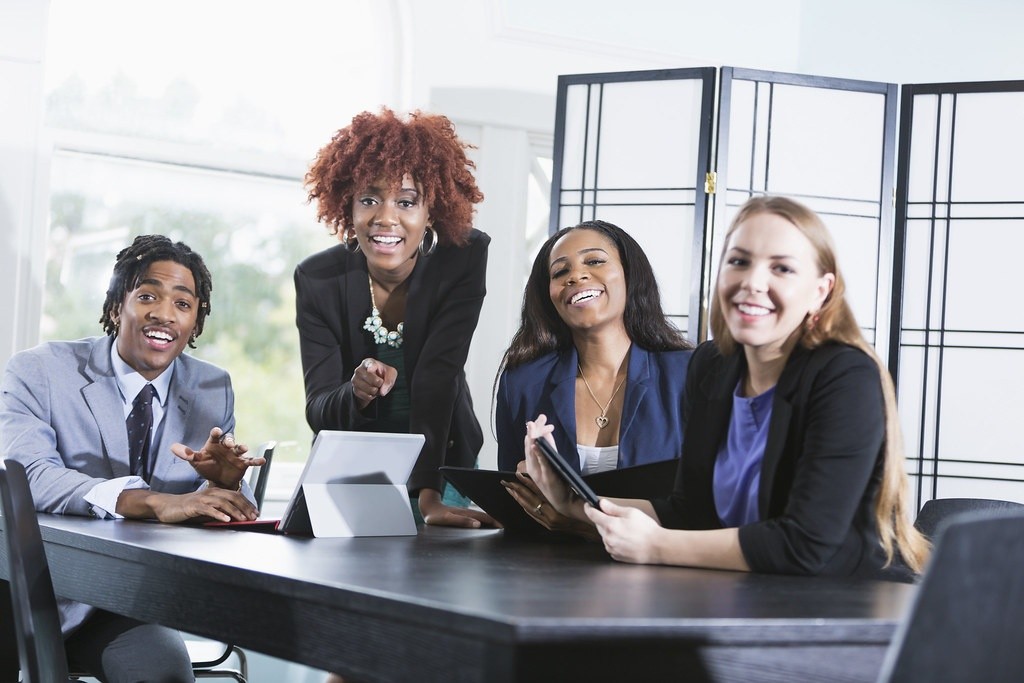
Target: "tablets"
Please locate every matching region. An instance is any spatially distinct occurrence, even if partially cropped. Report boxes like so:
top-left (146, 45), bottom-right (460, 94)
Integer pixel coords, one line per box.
top-left (278, 430), bottom-right (426, 535)
top-left (439, 466), bottom-right (570, 537)
top-left (536, 437), bottom-right (602, 512)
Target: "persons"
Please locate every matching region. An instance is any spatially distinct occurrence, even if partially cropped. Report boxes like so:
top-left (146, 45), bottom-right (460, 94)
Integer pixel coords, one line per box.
top-left (0, 235), bottom-right (266, 683)
top-left (490, 219), bottom-right (696, 543)
top-left (524, 197), bottom-right (937, 586)
top-left (294, 108), bottom-right (504, 683)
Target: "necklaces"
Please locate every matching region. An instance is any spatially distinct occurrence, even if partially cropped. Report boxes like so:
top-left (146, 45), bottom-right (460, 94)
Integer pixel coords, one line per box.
top-left (577, 363), bottom-right (627, 428)
top-left (362, 272), bottom-right (404, 349)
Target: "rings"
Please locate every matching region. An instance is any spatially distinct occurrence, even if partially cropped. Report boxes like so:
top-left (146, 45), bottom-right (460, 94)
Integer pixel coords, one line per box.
top-left (536, 502), bottom-right (543, 510)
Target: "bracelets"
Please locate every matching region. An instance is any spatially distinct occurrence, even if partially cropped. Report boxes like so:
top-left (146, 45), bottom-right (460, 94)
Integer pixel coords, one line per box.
top-left (204, 480), bottom-right (242, 492)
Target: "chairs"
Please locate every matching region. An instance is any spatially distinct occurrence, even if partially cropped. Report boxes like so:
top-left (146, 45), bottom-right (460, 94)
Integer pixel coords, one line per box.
top-left (69, 441), bottom-right (277, 683)
top-left (0, 459), bottom-right (70, 683)
top-left (878, 498), bottom-right (1024, 683)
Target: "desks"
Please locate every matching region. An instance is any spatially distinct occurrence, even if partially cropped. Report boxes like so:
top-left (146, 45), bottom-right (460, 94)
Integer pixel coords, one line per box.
top-left (38, 512), bottom-right (915, 683)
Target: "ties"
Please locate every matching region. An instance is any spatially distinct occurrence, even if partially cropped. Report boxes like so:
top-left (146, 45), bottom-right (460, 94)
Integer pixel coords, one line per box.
top-left (125, 382), bottom-right (156, 483)
